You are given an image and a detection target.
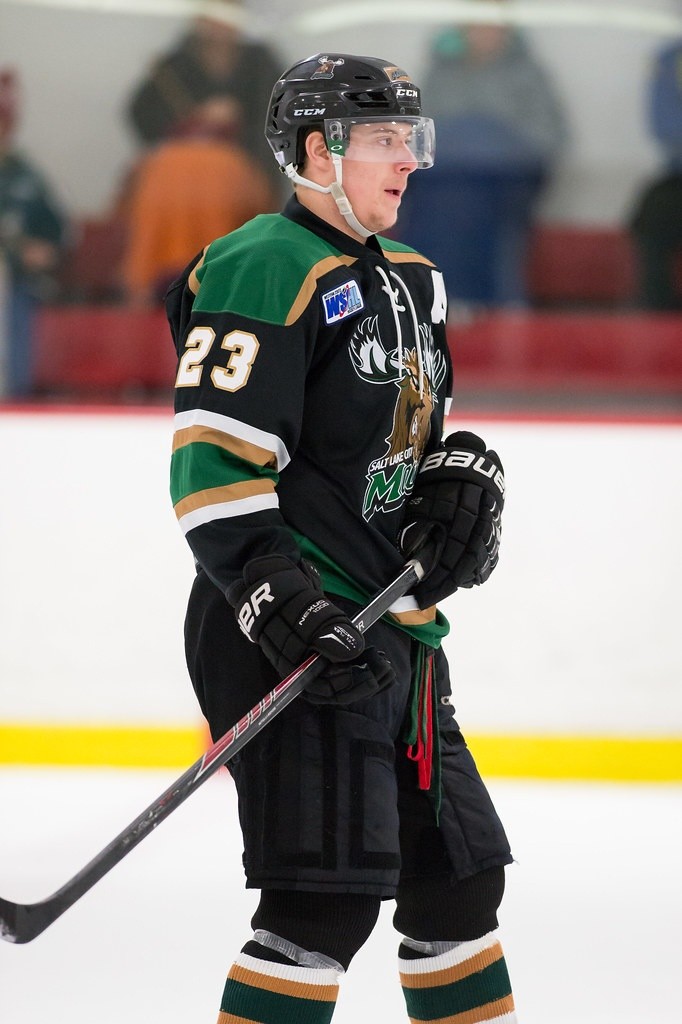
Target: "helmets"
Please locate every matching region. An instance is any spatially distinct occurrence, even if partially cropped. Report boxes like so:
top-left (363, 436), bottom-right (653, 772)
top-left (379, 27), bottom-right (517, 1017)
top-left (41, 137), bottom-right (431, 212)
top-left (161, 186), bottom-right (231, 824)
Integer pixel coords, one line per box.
top-left (264, 52), bottom-right (425, 173)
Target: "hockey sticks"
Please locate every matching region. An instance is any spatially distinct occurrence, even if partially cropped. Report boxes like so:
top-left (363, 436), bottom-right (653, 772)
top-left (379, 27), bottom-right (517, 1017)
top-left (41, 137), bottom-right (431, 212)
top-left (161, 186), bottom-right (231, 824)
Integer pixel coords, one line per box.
top-left (0, 544), bottom-right (439, 945)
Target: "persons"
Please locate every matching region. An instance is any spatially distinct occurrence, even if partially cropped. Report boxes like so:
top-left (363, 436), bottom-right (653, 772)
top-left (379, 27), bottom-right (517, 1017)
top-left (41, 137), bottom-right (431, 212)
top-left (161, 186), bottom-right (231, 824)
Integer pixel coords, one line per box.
top-left (165, 51), bottom-right (522, 1024)
top-left (1, 0), bottom-right (681, 401)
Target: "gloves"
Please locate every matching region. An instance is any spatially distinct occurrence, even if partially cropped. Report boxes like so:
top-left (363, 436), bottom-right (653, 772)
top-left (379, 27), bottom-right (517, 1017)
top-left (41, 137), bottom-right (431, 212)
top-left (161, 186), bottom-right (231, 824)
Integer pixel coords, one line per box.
top-left (224, 553), bottom-right (398, 706)
top-left (395, 431), bottom-right (505, 611)
